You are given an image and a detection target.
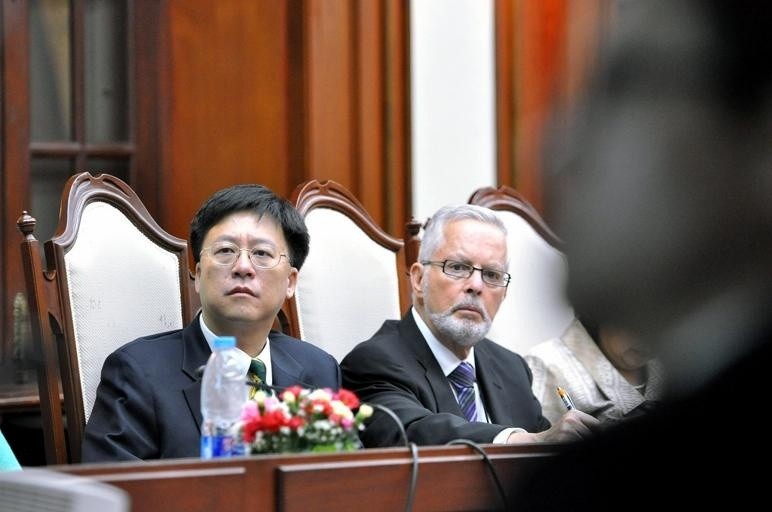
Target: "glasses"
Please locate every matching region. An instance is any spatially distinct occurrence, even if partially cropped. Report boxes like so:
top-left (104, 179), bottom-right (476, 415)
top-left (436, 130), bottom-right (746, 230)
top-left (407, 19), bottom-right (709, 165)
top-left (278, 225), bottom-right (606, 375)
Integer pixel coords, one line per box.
top-left (199, 242), bottom-right (292, 269)
top-left (421, 259), bottom-right (511, 288)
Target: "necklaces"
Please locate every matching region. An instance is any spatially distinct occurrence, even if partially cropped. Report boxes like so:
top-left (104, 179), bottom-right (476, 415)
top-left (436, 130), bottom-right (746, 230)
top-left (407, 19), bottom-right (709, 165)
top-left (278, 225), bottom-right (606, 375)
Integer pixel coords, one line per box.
top-left (630, 382), bottom-right (644, 391)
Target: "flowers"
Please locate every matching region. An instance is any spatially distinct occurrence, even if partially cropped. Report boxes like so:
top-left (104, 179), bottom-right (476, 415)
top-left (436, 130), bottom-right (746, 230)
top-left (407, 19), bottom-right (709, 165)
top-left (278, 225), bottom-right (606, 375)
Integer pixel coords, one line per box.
top-left (240, 384), bottom-right (375, 442)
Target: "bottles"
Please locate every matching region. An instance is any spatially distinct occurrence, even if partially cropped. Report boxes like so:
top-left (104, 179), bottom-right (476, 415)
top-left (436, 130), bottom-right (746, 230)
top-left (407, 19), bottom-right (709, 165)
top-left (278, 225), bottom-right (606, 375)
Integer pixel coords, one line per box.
top-left (198, 335), bottom-right (252, 461)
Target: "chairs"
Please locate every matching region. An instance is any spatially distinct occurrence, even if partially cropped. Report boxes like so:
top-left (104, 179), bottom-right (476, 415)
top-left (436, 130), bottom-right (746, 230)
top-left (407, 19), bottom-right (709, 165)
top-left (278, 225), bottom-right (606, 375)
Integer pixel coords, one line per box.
top-left (466, 182), bottom-right (569, 352)
top-left (15, 171), bottom-right (197, 466)
top-left (277, 178), bottom-right (423, 365)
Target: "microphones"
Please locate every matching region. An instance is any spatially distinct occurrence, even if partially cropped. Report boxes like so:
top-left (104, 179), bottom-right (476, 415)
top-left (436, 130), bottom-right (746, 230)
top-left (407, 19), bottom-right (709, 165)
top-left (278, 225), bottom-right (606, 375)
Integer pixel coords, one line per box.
top-left (195, 365), bottom-right (409, 447)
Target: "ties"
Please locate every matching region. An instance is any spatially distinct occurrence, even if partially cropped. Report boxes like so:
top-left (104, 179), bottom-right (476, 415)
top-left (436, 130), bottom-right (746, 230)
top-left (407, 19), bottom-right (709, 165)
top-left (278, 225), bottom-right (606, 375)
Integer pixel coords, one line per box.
top-left (247, 358), bottom-right (273, 399)
top-left (447, 363), bottom-right (477, 424)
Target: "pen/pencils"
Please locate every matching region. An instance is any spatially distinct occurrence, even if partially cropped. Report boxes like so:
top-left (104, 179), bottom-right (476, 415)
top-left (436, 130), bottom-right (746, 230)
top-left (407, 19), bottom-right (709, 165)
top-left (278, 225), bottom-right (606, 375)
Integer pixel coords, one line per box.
top-left (558, 386), bottom-right (575, 410)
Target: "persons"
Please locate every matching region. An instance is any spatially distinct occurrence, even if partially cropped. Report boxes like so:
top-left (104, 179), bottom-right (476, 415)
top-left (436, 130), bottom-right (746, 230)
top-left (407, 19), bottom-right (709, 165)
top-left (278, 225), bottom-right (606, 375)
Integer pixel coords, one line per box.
top-left (79, 185), bottom-right (342, 466)
top-left (525, 312), bottom-right (666, 429)
top-left (339, 204), bottom-right (603, 450)
